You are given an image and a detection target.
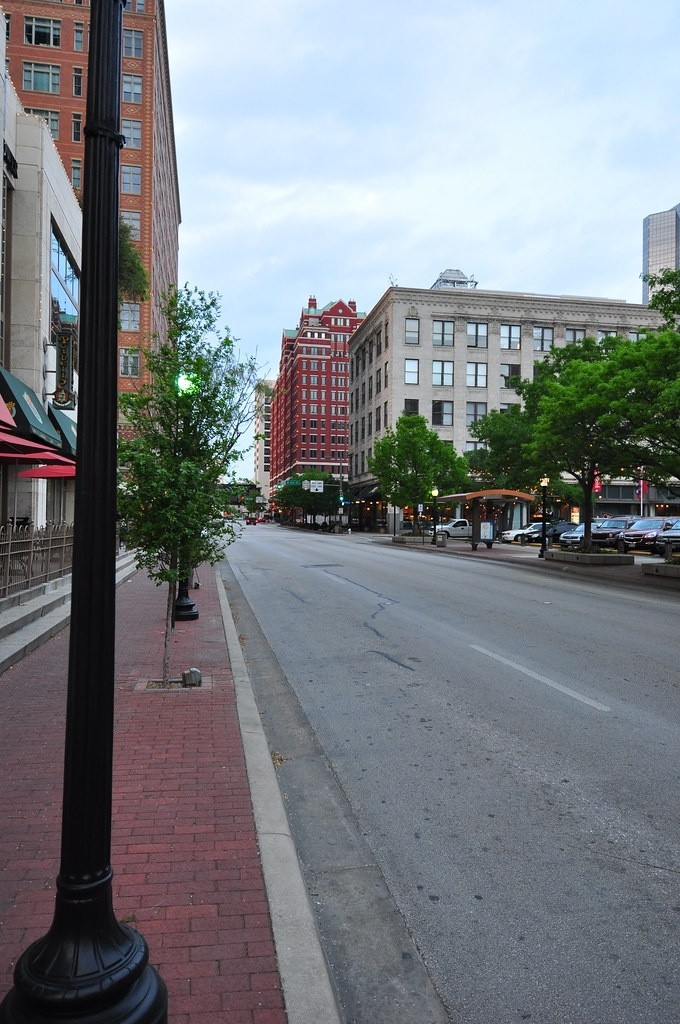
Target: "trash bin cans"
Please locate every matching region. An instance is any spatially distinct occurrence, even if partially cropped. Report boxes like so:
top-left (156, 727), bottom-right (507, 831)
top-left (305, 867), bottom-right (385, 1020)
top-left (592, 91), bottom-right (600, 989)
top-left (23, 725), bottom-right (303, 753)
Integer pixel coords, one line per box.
top-left (435, 532), bottom-right (447, 547)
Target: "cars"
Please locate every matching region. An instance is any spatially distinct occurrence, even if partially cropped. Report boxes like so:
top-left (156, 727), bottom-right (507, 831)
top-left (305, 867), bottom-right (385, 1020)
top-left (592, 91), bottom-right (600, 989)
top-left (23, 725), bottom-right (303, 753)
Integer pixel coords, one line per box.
top-left (501, 523), bottom-right (551, 543)
top-left (524, 522), bottom-right (580, 546)
top-left (559, 513), bottom-right (680, 556)
top-left (429, 518), bottom-right (473, 538)
top-left (246, 517), bottom-right (257, 525)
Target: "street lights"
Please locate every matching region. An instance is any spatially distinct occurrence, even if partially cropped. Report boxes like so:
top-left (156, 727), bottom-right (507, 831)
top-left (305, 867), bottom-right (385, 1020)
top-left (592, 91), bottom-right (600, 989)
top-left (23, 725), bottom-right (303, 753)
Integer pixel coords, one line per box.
top-left (537, 472), bottom-right (551, 558)
top-left (172, 358), bottom-right (200, 623)
top-left (431, 485), bottom-right (439, 545)
